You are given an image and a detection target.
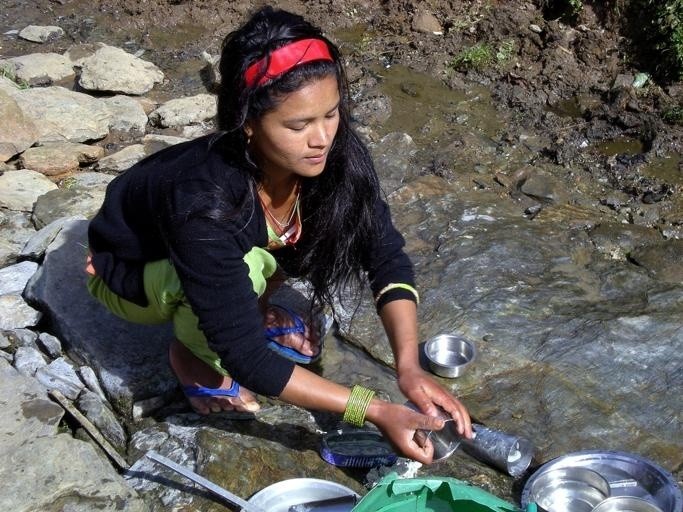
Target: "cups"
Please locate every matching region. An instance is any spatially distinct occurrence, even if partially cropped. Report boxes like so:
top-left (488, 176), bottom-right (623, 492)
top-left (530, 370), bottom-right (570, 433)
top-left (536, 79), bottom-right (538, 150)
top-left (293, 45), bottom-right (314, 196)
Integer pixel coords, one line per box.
top-left (461, 421), bottom-right (533, 477)
top-left (398, 396), bottom-right (465, 465)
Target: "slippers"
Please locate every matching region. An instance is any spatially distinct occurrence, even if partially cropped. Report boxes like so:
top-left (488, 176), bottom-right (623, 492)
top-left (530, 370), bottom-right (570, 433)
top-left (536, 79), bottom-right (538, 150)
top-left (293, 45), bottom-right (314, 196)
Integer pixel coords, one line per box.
top-left (166, 336), bottom-right (255, 421)
top-left (262, 302), bottom-right (322, 364)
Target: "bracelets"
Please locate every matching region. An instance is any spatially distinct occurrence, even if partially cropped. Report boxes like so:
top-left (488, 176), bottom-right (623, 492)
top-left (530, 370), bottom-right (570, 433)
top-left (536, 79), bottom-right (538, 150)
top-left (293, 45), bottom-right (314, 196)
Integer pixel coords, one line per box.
top-left (342, 383), bottom-right (376, 429)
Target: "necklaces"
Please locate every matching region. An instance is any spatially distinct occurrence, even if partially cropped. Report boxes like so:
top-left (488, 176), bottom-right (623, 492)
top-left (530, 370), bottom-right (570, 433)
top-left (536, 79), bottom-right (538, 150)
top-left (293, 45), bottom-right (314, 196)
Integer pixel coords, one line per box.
top-left (255, 189), bottom-right (300, 229)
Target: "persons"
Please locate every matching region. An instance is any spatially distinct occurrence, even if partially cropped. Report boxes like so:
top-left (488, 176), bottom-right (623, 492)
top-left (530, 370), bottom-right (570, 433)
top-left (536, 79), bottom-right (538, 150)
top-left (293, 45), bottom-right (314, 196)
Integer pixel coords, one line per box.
top-left (79, 5), bottom-right (475, 466)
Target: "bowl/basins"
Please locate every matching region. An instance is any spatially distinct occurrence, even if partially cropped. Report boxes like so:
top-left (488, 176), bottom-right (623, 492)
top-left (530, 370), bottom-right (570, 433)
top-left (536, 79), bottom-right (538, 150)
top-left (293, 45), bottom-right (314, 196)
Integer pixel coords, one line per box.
top-left (424, 334), bottom-right (476, 381)
top-left (520, 449), bottom-right (683, 512)
